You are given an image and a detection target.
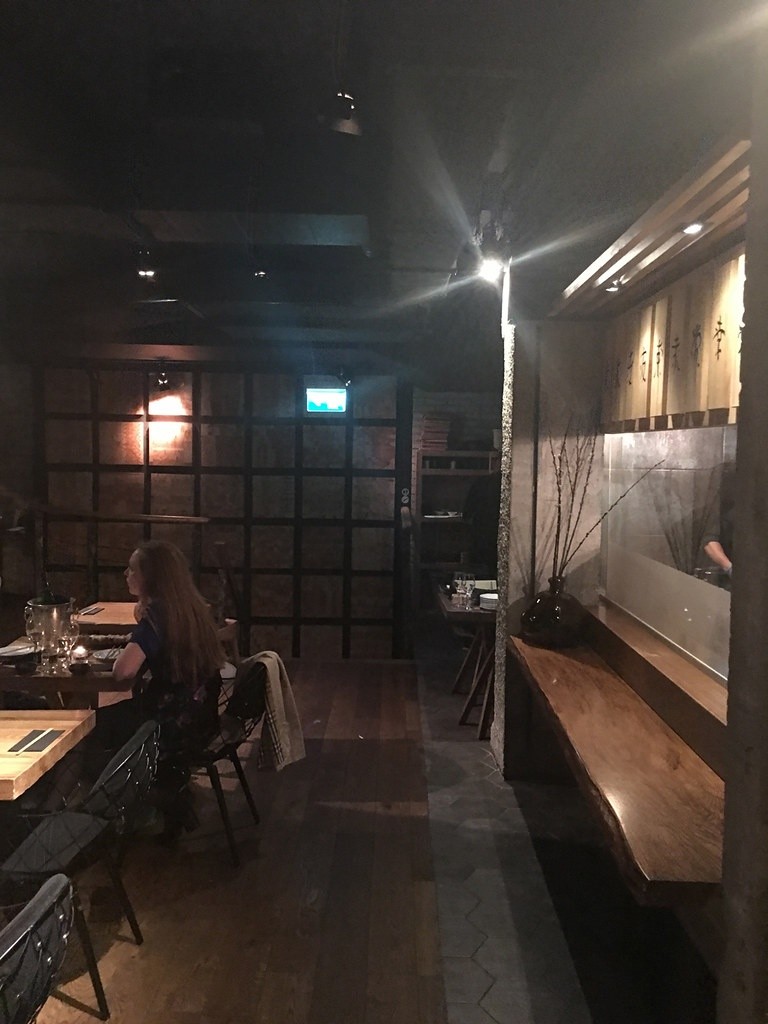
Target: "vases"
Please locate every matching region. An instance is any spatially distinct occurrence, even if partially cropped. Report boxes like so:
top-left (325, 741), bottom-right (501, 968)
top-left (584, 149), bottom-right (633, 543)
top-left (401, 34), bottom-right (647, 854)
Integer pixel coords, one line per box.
top-left (520, 577), bottom-right (591, 650)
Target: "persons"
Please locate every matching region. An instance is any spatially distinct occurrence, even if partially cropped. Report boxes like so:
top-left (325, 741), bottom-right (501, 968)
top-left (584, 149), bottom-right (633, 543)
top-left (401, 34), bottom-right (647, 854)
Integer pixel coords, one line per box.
top-left (458, 436), bottom-right (500, 580)
top-left (697, 460), bottom-right (733, 587)
top-left (112, 538), bottom-right (224, 845)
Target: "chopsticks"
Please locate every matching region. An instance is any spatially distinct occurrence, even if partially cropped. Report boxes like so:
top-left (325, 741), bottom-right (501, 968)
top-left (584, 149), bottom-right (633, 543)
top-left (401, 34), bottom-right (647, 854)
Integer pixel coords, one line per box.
top-left (104, 645), bottom-right (122, 661)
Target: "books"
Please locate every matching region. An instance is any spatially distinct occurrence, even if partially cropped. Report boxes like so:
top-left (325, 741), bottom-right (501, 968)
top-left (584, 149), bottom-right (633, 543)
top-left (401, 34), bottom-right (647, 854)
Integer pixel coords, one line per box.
top-left (419, 414), bottom-right (451, 451)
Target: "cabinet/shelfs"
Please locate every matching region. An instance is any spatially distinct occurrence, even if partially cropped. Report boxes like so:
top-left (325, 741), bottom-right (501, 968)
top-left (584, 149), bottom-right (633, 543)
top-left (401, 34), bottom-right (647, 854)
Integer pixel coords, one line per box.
top-left (417, 448), bottom-right (504, 620)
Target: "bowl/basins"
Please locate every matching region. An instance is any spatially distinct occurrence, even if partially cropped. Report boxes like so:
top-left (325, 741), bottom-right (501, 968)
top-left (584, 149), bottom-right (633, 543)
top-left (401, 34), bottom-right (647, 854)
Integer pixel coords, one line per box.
top-left (436, 512), bottom-right (445, 515)
top-left (15, 661), bottom-right (37, 675)
top-left (447, 512), bottom-right (458, 515)
top-left (69, 663), bottom-right (90, 675)
top-left (452, 593), bottom-right (466, 604)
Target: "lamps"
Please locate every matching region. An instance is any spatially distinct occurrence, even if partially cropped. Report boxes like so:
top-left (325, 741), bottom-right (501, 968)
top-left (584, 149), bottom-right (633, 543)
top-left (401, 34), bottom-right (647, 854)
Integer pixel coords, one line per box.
top-left (317, 83), bottom-right (368, 140)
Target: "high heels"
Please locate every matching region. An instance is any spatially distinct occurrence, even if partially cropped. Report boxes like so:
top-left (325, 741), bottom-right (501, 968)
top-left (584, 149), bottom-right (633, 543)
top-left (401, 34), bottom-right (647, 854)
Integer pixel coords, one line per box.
top-left (160, 794), bottom-right (201, 840)
top-left (156, 778), bottom-right (195, 817)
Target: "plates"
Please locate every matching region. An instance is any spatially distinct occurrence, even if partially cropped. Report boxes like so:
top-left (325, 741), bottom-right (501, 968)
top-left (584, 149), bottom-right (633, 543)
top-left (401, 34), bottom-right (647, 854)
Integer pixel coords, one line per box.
top-left (93, 648), bottom-right (125, 660)
top-left (424, 515), bottom-right (460, 519)
top-left (479, 593), bottom-right (498, 610)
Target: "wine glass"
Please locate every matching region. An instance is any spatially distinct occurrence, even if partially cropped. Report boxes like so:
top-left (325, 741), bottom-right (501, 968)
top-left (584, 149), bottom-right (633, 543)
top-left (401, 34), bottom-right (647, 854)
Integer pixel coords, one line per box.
top-left (25, 616), bottom-right (48, 653)
top-left (51, 621), bottom-right (80, 667)
top-left (452, 571), bottom-right (467, 607)
top-left (460, 574), bottom-right (476, 611)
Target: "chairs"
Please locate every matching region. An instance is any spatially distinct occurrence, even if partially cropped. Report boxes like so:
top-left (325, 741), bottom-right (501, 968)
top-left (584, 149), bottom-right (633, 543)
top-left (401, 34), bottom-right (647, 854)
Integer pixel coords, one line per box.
top-left (451, 568), bottom-right (483, 648)
top-left (0, 647), bottom-right (306, 1024)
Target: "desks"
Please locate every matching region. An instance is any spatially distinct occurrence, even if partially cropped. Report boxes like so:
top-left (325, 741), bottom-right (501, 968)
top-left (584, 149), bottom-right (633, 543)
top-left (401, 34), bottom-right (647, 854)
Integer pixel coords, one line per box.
top-left (430, 583), bottom-right (497, 743)
top-left (69, 601), bottom-right (142, 636)
top-left (0, 709), bottom-right (96, 801)
top-left (509, 630), bottom-right (727, 909)
top-left (0, 634), bottom-right (146, 710)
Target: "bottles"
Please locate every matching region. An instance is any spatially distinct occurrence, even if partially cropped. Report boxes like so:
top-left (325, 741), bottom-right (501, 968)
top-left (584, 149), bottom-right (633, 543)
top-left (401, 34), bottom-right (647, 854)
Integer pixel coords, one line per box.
top-left (39, 568), bottom-right (58, 604)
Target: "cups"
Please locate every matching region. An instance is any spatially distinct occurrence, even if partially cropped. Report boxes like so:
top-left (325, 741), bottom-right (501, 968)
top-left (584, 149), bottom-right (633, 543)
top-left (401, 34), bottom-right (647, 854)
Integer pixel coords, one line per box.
top-left (71, 650), bottom-right (89, 664)
top-left (41, 653), bottom-right (58, 674)
